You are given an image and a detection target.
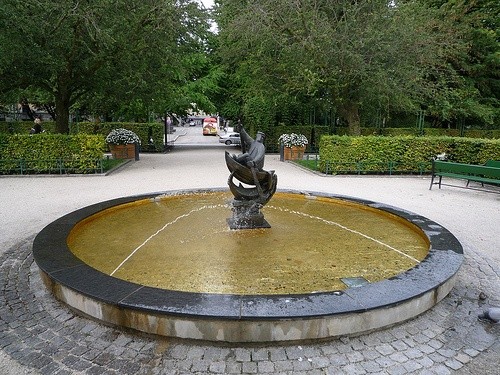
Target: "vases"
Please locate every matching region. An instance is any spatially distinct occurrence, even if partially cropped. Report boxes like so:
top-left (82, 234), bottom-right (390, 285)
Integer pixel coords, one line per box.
top-left (284, 146), bottom-right (306, 160)
top-left (108, 144), bottom-right (136, 159)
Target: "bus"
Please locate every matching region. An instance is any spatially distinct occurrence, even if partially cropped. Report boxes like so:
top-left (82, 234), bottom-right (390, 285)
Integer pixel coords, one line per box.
top-left (202, 117), bottom-right (217, 135)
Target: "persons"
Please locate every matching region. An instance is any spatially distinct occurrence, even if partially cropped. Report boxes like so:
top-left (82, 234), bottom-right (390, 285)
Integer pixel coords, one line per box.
top-left (31, 118), bottom-right (42, 134)
top-left (232, 124), bottom-right (266, 170)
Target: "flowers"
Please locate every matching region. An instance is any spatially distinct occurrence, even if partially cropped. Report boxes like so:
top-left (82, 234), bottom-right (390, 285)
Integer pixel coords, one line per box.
top-left (278, 133), bottom-right (308, 147)
top-left (106, 128), bottom-right (141, 144)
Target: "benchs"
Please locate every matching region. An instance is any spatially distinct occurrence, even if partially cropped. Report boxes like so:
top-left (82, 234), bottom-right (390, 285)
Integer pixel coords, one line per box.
top-left (428, 156), bottom-right (500, 190)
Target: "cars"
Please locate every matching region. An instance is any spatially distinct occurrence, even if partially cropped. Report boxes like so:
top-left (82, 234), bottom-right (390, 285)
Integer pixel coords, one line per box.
top-left (219, 132), bottom-right (241, 145)
top-left (190, 121), bottom-right (196, 126)
